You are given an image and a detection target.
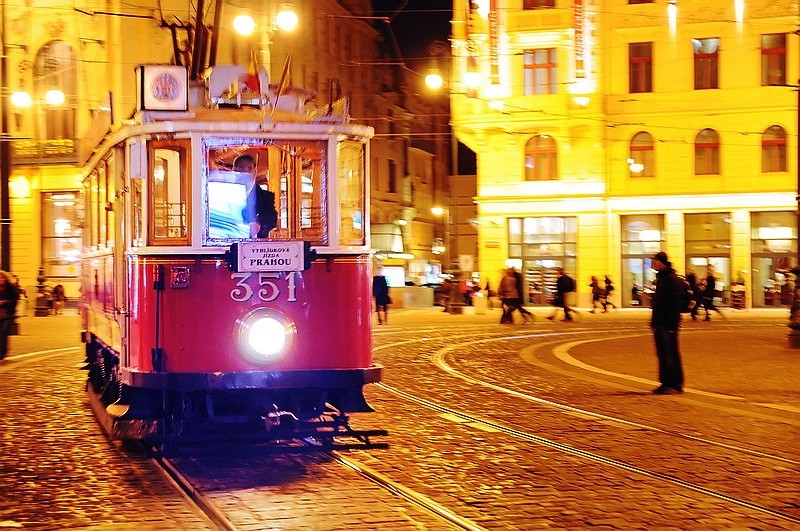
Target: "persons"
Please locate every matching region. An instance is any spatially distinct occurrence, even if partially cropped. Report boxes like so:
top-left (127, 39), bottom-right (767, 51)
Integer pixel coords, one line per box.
top-left (372, 265), bottom-right (393, 325)
top-left (631, 252), bottom-right (728, 396)
top-left (589, 274), bottom-right (618, 313)
top-left (553, 267), bottom-right (576, 321)
top-left (52, 279), bottom-right (65, 316)
top-left (0, 270), bottom-right (27, 362)
top-left (497, 266), bottom-right (537, 325)
top-left (230, 154), bottom-right (277, 239)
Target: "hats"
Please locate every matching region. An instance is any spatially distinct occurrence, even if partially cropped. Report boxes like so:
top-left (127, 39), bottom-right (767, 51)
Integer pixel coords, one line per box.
top-left (650, 252), bottom-right (667, 259)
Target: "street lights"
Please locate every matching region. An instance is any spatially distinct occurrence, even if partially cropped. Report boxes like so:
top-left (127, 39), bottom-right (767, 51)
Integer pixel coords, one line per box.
top-left (231, 11), bottom-right (298, 231)
top-left (424, 59), bottom-right (480, 314)
top-left (10, 87), bottom-right (66, 316)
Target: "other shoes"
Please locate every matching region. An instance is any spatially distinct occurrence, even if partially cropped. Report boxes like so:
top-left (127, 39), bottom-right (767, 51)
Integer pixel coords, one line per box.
top-left (652, 382), bottom-right (672, 395)
top-left (671, 384), bottom-right (683, 394)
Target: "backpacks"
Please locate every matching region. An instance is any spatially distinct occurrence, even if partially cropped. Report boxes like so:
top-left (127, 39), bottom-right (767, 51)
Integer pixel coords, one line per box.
top-left (670, 269), bottom-right (696, 314)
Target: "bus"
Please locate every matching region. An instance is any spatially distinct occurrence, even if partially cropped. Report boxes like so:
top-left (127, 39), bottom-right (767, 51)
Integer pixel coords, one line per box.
top-left (78, 0), bottom-right (390, 463)
